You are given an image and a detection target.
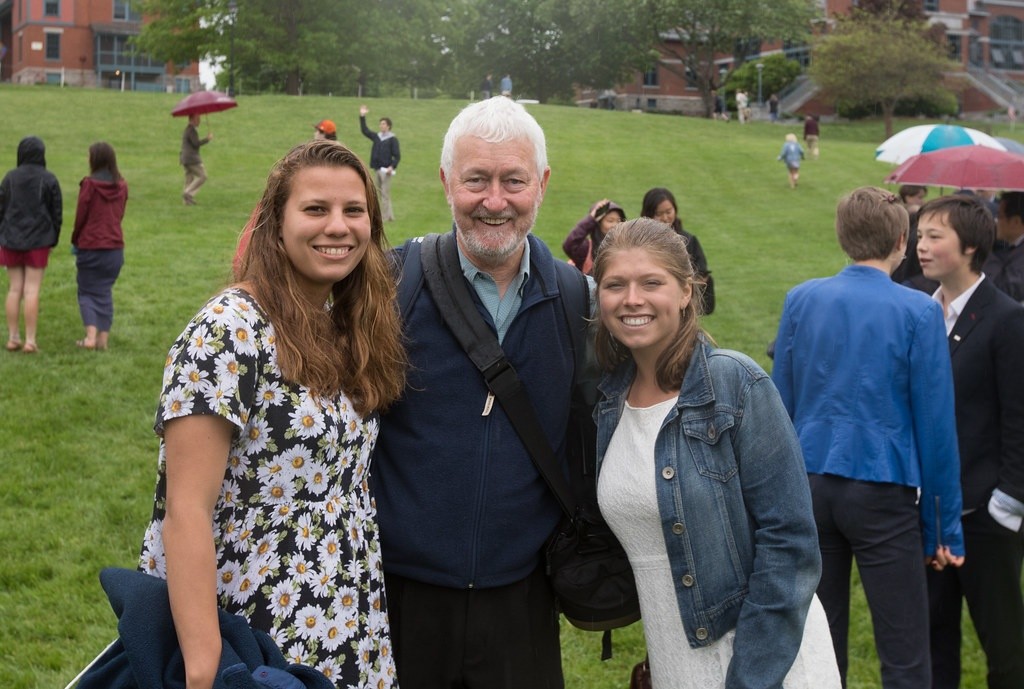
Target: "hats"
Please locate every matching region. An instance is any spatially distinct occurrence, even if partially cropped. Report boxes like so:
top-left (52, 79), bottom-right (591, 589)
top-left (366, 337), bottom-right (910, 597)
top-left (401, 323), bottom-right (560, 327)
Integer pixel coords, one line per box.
top-left (313, 119), bottom-right (336, 136)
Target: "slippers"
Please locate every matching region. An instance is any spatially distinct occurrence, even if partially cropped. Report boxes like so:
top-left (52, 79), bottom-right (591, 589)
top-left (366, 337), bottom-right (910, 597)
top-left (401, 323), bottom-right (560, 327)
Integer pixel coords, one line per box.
top-left (74, 338), bottom-right (97, 351)
top-left (95, 344), bottom-right (108, 351)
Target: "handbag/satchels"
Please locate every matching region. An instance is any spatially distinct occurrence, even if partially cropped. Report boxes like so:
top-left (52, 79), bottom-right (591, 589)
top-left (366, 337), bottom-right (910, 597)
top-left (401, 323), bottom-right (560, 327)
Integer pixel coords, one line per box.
top-left (541, 497), bottom-right (641, 630)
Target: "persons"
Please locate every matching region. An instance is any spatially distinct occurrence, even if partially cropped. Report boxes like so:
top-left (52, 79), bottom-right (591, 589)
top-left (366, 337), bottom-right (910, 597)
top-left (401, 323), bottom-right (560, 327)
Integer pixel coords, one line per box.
top-left (136, 142), bottom-right (408, 689)
top-left (639, 188), bottom-right (715, 317)
top-left (593, 218), bottom-right (844, 689)
top-left (996, 192), bottom-right (1024, 302)
top-left (180, 113), bottom-right (213, 207)
top-left (358, 102), bottom-right (400, 222)
top-left (777, 132), bottom-right (807, 189)
top-left (308, 119), bottom-right (338, 141)
top-left (478, 72), bottom-right (514, 100)
top-left (367, 93), bottom-right (598, 689)
top-left (0, 133), bottom-right (63, 355)
top-left (562, 199), bottom-right (627, 278)
top-left (709, 89), bottom-right (778, 125)
top-left (70, 141), bottom-right (128, 352)
top-left (900, 184), bottom-right (928, 216)
top-left (915, 194), bottom-right (1024, 689)
top-left (768, 188), bottom-right (965, 689)
top-left (802, 112), bottom-right (819, 163)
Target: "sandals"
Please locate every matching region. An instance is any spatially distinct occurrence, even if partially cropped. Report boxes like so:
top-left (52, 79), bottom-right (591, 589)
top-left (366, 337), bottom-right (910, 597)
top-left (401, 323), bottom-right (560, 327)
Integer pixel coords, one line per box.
top-left (23, 342), bottom-right (38, 354)
top-left (6, 340), bottom-right (22, 351)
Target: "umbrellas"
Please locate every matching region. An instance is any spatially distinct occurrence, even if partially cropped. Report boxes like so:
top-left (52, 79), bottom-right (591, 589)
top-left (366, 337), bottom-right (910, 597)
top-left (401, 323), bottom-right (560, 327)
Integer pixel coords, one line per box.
top-left (882, 144), bottom-right (1024, 190)
top-left (170, 90), bottom-right (238, 133)
top-left (874, 123), bottom-right (1024, 166)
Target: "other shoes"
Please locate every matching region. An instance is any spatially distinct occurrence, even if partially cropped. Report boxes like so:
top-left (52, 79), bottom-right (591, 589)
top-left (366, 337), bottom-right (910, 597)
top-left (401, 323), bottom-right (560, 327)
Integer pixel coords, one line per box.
top-left (182, 191), bottom-right (197, 205)
